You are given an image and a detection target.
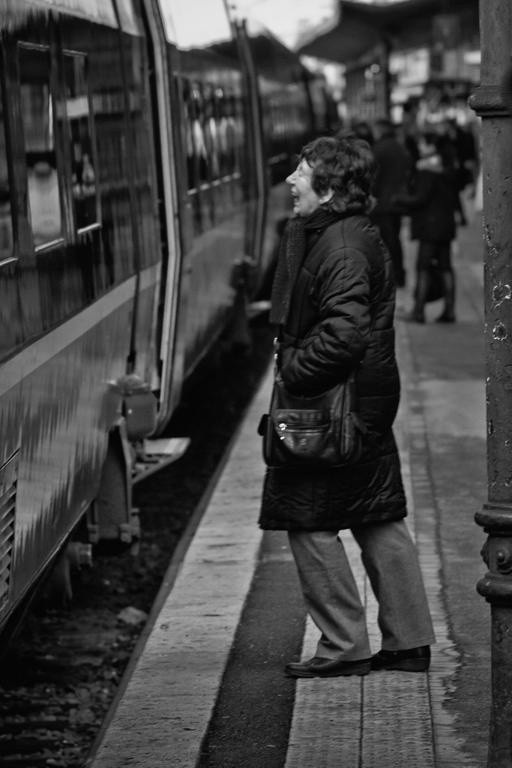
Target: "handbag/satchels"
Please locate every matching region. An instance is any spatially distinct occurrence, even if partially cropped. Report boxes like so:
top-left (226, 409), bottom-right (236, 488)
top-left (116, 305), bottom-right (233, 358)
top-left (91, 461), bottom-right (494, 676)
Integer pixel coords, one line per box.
top-left (256, 351), bottom-right (372, 470)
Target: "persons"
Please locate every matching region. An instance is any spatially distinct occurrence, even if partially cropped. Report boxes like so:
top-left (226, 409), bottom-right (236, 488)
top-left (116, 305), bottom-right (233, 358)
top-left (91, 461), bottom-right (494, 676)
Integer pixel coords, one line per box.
top-left (313, 104), bottom-right (483, 330)
top-left (224, 131), bottom-right (435, 685)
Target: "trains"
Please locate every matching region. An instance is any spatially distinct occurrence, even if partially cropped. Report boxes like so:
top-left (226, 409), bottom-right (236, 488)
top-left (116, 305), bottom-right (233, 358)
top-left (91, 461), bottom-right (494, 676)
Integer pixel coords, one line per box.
top-left (0, 0), bottom-right (338, 651)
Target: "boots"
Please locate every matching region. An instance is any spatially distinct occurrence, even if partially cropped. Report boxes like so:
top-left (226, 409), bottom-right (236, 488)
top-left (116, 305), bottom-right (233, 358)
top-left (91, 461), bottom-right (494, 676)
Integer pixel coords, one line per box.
top-left (434, 303), bottom-right (456, 323)
top-left (397, 303), bottom-right (426, 325)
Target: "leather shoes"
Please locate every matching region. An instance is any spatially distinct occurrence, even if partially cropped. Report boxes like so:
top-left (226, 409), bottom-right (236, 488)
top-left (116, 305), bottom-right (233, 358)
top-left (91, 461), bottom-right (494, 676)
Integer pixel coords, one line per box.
top-left (371, 644), bottom-right (431, 672)
top-left (284, 656), bottom-right (371, 678)
